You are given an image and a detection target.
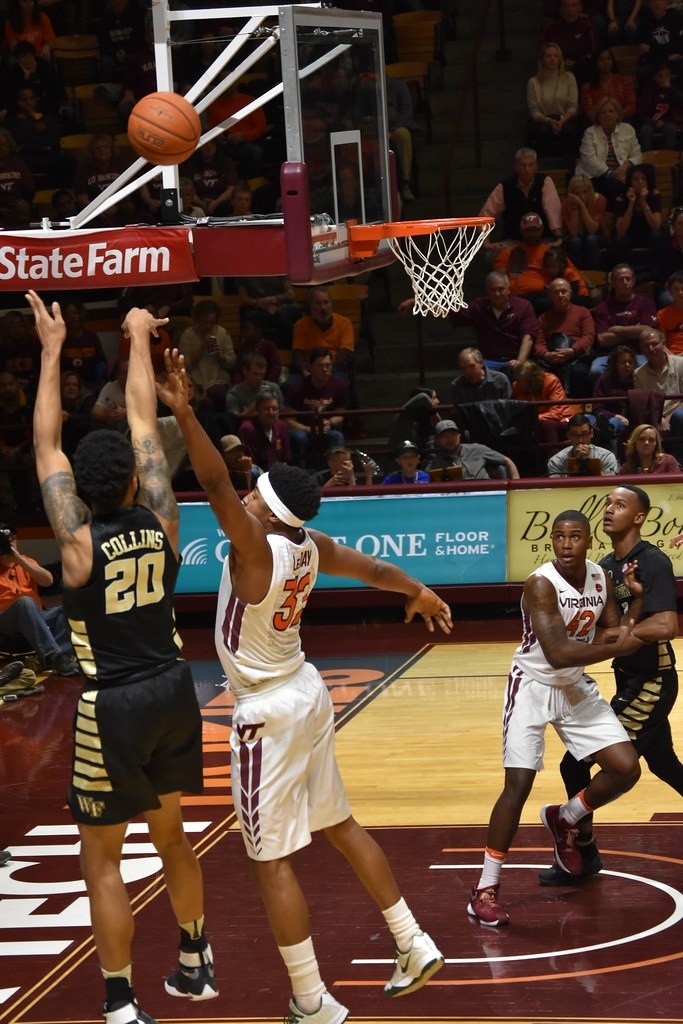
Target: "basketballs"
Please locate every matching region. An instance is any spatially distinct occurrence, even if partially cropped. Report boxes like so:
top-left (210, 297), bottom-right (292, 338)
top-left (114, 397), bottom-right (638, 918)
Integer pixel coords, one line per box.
top-left (127, 91), bottom-right (202, 166)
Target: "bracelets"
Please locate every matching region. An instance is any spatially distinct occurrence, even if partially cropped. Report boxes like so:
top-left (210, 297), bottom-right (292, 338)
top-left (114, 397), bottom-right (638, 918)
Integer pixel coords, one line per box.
top-left (609, 19), bottom-right (616, 23)
top-left (624, 161), bottom-right (632, 167)
top-left (572, 208), bottom-right (578, 210)
top-left (545, 117), bottom-right (550, 125)
top-left (643, 208), bottom-right (650, 213)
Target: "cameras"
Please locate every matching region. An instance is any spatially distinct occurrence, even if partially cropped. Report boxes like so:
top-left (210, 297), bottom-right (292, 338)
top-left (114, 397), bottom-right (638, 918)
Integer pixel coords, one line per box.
top-left (0, 529), bottom-right (12, 555)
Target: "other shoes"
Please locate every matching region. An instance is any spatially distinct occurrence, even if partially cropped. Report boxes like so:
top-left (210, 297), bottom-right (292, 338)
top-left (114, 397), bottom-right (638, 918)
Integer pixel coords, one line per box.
top-left (0, 661), bottom-right (24, 683)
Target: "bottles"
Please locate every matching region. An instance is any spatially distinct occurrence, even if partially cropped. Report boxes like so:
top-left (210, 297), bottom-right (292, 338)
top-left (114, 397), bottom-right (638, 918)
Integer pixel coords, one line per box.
top-left (353, 449), bottom-right (381, 475)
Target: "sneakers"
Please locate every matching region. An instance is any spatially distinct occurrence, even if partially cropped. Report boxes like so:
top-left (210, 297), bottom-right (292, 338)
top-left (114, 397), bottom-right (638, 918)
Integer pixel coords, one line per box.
top-left (466, 881), bottom-right (510, 926)
top-left (382, 931), bottom-right (445, 998)
top-left (285, 992), bottom-right (350, 1024)
top-left (100, 976), bottom-right (159, 1024)
top-left (539, 838), bottom-right (603, 885)
top-left (164, 937), bottom-right (220, 1001)
top-left (540, 805), bottom-right (581, 876)
top-left (52, 655), bottom-right (80, 674)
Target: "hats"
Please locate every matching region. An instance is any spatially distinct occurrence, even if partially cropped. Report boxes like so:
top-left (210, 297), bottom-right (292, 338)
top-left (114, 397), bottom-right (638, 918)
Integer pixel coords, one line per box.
top-left (436, 420), bottom-right (457, 432)
top-left (519, 212), bottom-right (546, 229)
top-left (396, 440), bottom-right (419, 454)
top-left (220, 436), bottom-right (243, 450)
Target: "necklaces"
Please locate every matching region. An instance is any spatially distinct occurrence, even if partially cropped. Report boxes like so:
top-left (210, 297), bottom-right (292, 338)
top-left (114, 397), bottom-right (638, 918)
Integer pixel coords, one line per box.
top-left (643, 467), bottom-right (649, 470)
top-left (541, 73), bottom-right (559, 114)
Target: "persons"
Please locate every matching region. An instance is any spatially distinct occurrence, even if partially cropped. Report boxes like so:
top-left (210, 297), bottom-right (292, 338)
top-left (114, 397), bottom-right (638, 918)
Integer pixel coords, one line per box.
top-left (0, 523), bottom-right (80, 687)
top-left (154, 347), bottom-right (454, 1024)
top-left (25, 288), bottom-right (220, 1024)
top-left (539, 484), bottom-right (683, 885)
top-left (467, 511), bottom-right (650, 927)
top-left (0, 0), bottom-right (683, 506)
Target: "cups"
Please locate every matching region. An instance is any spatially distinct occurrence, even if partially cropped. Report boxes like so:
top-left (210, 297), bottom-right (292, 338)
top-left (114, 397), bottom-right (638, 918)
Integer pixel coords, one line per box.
top-left (205, 335), bottom-right (218, 355)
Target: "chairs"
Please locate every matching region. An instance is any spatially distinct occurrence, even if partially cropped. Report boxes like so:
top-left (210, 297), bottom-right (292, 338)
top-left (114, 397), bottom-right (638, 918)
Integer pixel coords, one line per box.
top-left (2, 12), bottom-right (683, 359)
